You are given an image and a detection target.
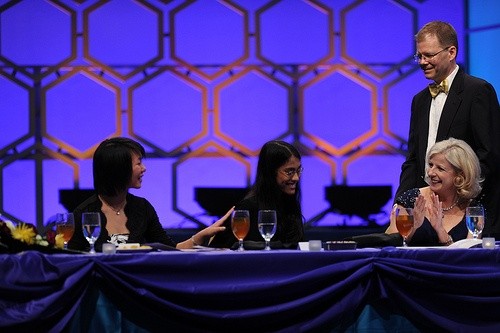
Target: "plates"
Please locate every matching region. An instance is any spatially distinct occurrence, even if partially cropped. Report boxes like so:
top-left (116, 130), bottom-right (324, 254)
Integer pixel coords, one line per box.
top-left (116, 246), bottom-right (153, 253)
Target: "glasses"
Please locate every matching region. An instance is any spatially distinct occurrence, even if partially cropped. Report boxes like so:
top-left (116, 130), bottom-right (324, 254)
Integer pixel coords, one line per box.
top-left (280, 167), bottom-right (303, 178)
top-left (414, 45), bottom-right (453, 63)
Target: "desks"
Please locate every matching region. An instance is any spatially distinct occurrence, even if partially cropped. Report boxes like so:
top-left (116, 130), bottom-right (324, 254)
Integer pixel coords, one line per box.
top-left (1, 245), bottom-right (500, 333)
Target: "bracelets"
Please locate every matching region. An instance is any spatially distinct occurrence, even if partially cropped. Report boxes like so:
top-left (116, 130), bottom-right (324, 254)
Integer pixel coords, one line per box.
top-left (439, 235), bottom-right (453, 246)
top-left (191, 235), bottom-right (201, 246)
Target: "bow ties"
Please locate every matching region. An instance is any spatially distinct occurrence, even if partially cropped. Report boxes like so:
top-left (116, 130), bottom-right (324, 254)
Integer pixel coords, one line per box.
top-left (428, 80), bottom-right (448, 98)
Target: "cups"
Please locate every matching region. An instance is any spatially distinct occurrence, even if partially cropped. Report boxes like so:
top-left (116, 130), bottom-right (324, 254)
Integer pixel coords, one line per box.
top-left (102, 243), bottom-right (116, 255)
top-left (308, 240), bottom-right (323, 251)
top-left (482, 237), bottom-right (496, 249)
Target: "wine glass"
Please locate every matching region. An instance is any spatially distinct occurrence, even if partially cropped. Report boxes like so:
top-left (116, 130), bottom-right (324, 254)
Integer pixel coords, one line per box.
top-left (257, 209), bottom-right (278, 250)
top-left (55, 213), bottom-right (76, 250)
top-left (396, 208), bottom-right (414, 247)
top-left (231, 210), bottom-right (250, 252)
top-left (81, 212), bottom-right (102, 254)
top-left (466, 206), bottom-right (485, 249)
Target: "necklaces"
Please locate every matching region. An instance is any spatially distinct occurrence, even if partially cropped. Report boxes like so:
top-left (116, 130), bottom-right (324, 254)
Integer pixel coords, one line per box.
top-left (100, 192), bottom-right (124, 215)
top-left (443, 198), bottom-right (459, 211)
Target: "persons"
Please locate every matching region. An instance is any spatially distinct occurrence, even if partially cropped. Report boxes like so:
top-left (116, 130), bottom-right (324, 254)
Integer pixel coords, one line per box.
top-left (68, 137), bottom-right (236, 250)
top-left (386, 137), bottom-right (499, 247)
top-left (219, 140), bottom-right (305, 250)
top-left (394, 21), bottom-right (500, 204)
